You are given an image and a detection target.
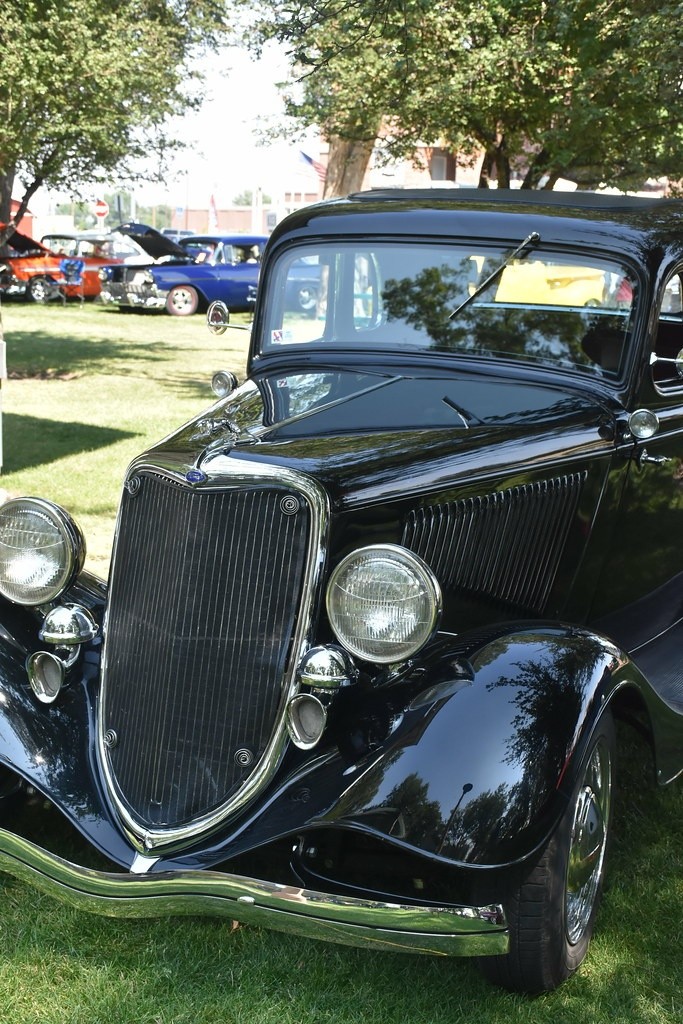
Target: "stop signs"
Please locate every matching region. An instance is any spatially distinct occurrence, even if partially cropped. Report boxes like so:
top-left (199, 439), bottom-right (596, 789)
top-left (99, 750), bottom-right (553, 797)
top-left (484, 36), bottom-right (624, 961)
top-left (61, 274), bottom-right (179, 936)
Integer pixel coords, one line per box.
top-left (94, 201), bottom-right (109, 219)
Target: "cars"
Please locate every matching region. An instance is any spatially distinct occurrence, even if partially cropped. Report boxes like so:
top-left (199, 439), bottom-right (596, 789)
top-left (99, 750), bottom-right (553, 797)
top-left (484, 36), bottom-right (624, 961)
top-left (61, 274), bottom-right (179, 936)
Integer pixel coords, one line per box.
top-left (39, 223), bottom-right (197, 261)
top-left (1, 223), bottom-right (125, 305)
top-left (97, 222), bottom-right (324, 317)
top-left (0, 185), bottom-right (682, 996)
top-left (468, 254), bottom-right (683, 316)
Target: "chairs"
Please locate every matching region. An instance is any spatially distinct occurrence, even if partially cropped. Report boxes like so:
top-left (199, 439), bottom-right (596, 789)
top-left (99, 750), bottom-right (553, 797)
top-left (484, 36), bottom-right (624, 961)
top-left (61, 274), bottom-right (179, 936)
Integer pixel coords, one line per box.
top-left (43, 258), bottom-right (86, 308)
